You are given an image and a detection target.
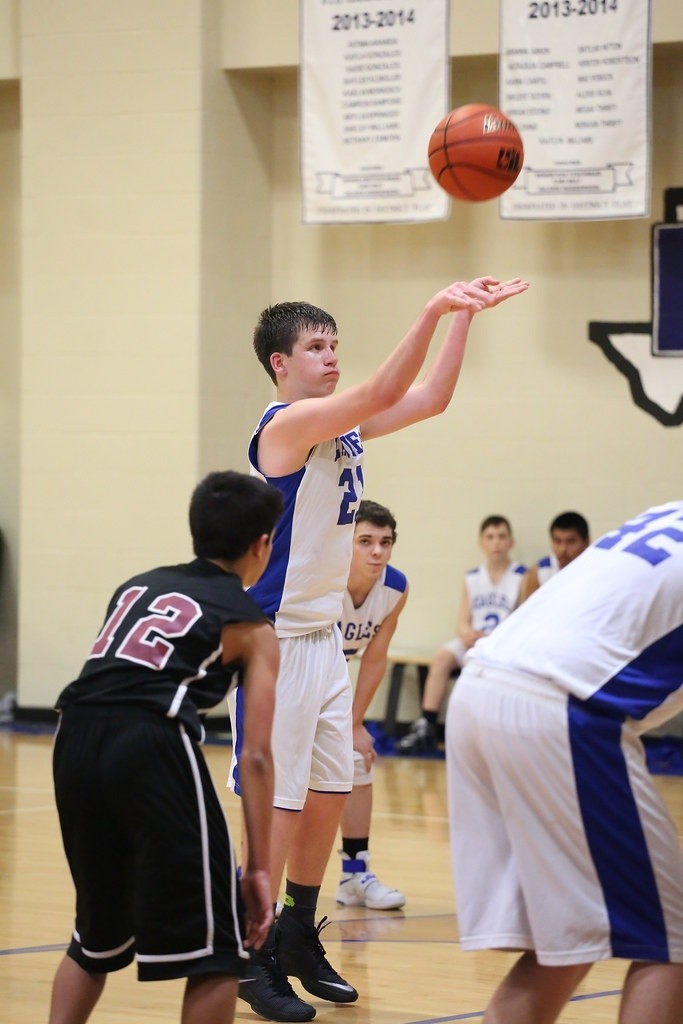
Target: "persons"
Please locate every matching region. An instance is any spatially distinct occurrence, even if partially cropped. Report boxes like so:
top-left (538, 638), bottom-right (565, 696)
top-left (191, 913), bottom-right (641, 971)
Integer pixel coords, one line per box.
top-left (233, 276), bottom-right (530, 1022)
top-left (398, 512), bottom-right (589, 751)
top-left (49, 469), bottom-right (283, 1024)
top-left (446, 500), bottom-right (683, 1024)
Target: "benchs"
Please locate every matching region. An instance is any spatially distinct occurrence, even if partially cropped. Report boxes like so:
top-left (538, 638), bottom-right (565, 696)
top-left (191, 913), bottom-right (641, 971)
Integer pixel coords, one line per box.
top-left (383, 654), bottom-right (462, 751)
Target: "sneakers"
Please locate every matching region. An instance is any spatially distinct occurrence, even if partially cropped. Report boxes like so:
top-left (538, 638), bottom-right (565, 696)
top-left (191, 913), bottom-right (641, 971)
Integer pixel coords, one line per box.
top-left (237, 940), bottom-right (317, 1023)
top-left (335, 849), bottom-right (406, 910)
top-left (265, 911), bottom-right (358, 1004)
top-left (237, 869), bottom-right (284, 918)
top-left (394, 718), bottom-right (438, 758)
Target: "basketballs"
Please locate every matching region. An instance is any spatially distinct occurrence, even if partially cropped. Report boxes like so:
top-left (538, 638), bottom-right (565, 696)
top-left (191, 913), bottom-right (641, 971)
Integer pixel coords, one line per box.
top-left (425, 102), bottom-right (526, 204)
